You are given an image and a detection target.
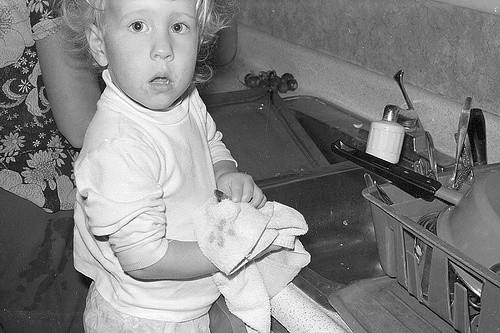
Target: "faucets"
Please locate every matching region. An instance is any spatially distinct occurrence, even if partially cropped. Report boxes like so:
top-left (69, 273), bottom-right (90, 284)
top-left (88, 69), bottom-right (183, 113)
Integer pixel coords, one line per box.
top-left (365, 103), bottom-right (419, 165)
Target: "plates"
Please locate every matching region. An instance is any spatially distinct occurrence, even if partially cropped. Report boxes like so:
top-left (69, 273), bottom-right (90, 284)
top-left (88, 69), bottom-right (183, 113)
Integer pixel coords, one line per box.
top-left (414, 212), bottom-right (439, 258)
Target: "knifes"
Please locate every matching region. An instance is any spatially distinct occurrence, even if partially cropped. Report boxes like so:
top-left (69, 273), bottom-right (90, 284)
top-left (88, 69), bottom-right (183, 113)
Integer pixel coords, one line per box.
top-left (375, 181), bottom-right (393, 206)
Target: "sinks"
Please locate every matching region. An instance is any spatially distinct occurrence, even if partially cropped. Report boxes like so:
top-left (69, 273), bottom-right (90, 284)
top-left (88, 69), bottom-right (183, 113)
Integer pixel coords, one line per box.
top-left (201, 106), bottom-right (365, 182)
top-left (259, 164), bottom-right (432, 312)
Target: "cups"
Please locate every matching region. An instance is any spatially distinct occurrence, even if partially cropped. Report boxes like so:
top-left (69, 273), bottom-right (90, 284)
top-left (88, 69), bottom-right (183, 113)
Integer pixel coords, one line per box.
top-left (402, 111), bottom-right (429, 151)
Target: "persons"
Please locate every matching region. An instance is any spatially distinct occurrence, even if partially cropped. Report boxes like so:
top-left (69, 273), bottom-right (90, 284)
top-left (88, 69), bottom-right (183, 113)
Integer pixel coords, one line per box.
top-left (0, 0), bottom-right (102, 332)
top-left (73, 0), bottom-right (283, 332)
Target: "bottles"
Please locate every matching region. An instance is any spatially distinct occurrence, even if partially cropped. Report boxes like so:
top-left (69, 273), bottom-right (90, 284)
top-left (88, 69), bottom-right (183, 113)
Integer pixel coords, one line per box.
top-left (364, 105), bottom-right (405, 164)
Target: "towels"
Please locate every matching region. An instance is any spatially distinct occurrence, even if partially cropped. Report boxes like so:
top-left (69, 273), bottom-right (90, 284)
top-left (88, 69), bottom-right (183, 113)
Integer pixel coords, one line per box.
top-left (191, 199), bottom-right (311, 333)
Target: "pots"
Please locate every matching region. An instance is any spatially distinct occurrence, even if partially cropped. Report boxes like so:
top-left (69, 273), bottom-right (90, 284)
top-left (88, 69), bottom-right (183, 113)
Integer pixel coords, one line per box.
top-left (331, 139), bottom-right (500, 297)
